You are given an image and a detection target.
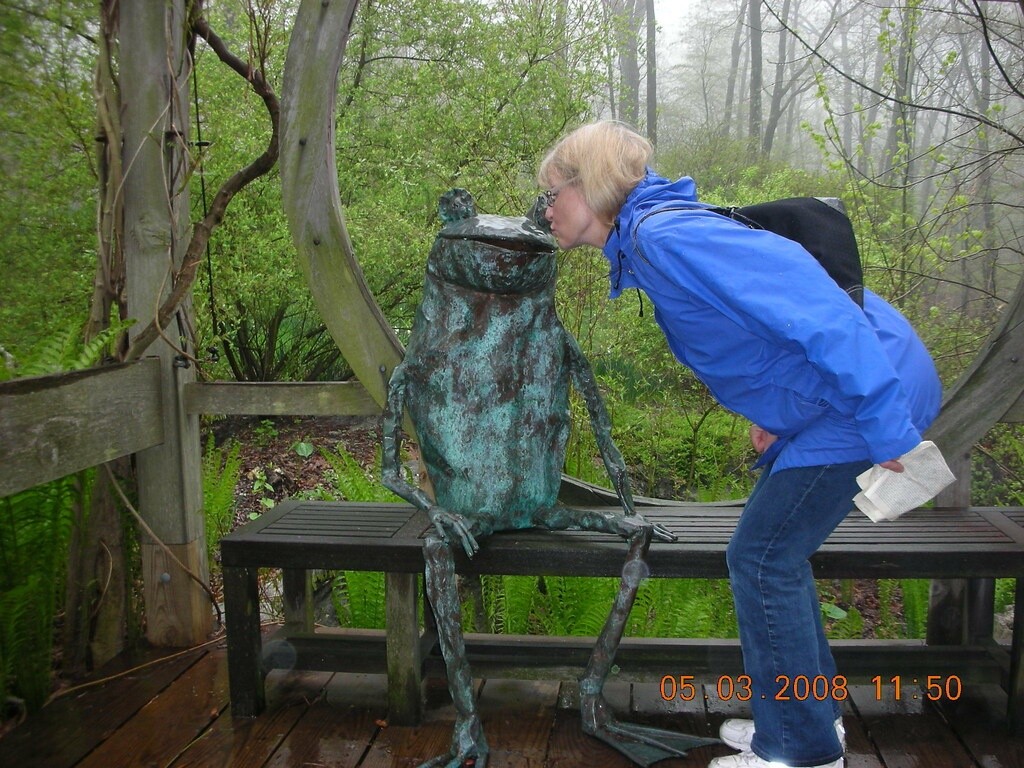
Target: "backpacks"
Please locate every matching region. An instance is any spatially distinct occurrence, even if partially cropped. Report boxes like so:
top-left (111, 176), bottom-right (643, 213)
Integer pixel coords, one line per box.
top-left (634, 196), bottom-right (864, 311)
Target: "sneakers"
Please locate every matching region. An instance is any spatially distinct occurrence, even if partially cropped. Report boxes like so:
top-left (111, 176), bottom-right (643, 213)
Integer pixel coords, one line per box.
top-left (719, 717), bottom-right (846, 760)
top-left (707, 750), bottom-right (843, 768)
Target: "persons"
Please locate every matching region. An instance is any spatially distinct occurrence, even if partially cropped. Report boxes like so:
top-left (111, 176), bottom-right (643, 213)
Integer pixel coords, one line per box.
top-left (538, 122), bottom-right (943, 768)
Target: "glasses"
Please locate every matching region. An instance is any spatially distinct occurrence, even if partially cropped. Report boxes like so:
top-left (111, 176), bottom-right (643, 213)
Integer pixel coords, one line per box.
top-left (543, 176), bottom-right (580, 207)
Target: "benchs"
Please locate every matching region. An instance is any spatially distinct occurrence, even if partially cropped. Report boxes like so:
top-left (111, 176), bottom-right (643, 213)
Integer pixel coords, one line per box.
top-left (219, 502), bottom-right (1024, 729)
